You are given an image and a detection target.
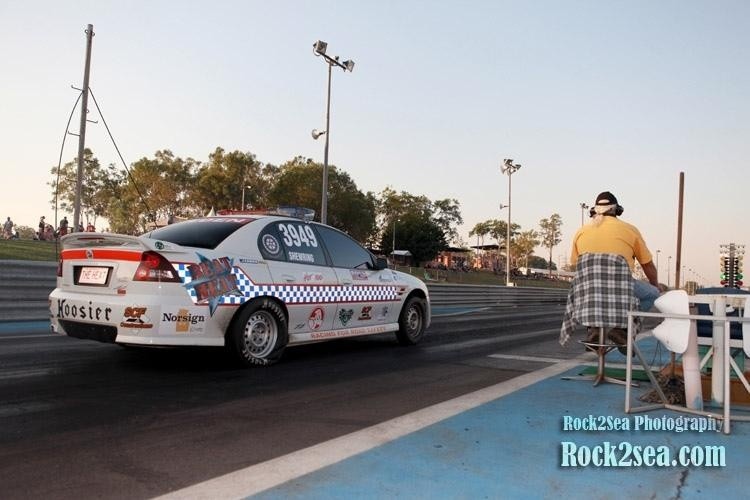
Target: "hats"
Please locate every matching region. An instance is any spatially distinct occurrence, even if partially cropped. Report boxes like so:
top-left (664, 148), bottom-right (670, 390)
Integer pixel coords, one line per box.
top-left (594, 191), bottom-right (617, 206)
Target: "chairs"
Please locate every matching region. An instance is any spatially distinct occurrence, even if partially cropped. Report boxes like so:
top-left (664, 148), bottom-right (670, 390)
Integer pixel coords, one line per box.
top-left (690, 286), bottom-right (750, 406)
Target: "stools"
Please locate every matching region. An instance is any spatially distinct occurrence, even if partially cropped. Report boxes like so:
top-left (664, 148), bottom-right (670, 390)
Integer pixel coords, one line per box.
top-left (567, 318), bottom-right (626, 387)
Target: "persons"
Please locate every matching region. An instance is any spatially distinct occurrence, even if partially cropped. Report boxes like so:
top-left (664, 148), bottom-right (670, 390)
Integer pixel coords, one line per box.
top-left (386, 254), bottom-right (394, 268)
top-left (0, 217), bottom-right (95, 242)
top-left (559, 192), bottom-right (663, 358)
top-left (421, 257), bottom-right (471, 271)
top-left (511, 267), bottom-right (541, 280)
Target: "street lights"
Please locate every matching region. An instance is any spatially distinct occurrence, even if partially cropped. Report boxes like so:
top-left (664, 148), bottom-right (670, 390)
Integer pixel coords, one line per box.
top-left (241, 185), bottom-right (252, 210)
top-left (655, 250), bottom-right (661, 270)
top-left (498, 158), bottom-right (521, 288)
top-left (392, 218), bottom-right (401, 264)
top-left (682, 265), bottom-right (704, 288)
top-left (667, 256), bottom-right (672, 286)
top-left (579, 202), bottom-right (589, 227)
top-left (310, 40), bottom-right (355, 227)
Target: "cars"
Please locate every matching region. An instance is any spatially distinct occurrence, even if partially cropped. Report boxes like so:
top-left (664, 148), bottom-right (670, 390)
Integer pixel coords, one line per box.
top-left (32, 226), bottom-right (75, 240)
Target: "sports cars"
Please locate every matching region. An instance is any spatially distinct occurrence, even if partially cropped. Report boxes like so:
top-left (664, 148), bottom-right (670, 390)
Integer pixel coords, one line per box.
top-left (47, 211), bottom-right (432, 373)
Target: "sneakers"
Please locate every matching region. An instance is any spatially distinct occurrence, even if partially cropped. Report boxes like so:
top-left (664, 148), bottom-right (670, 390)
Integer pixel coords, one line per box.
top-left (606, 326), bottom-right (636, 358)
top-left (584, 326), bottom-right (605, 352)
